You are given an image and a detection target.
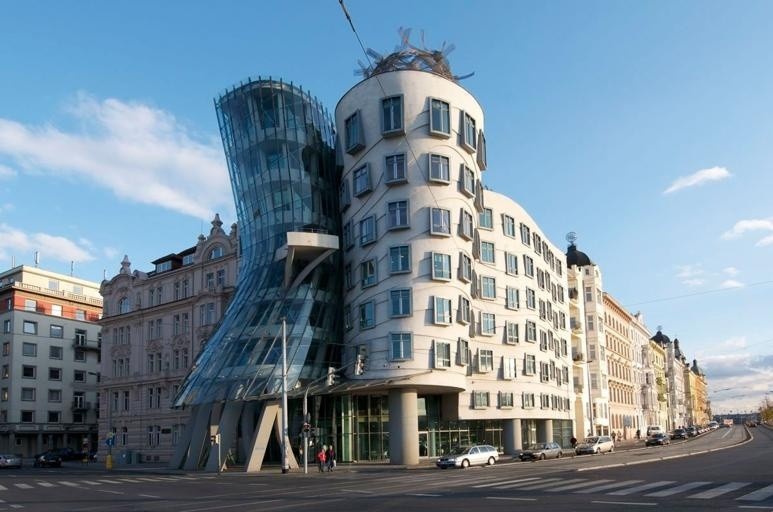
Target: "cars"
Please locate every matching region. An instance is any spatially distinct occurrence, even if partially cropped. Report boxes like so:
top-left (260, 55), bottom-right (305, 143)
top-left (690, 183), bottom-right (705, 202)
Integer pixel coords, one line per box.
top-left (518, 442), bottom-right (562, 462)
top-left (671, 421), bottom-right (719, 440)
top-left (576, 436), bottom-right (613, 455)
top-left (436, 445), bottom-right (498, 469)
top-left (648, 425), bottom-right (662, 435)
top-left (645, 435), bottom-right (671, 447)
top-left (34, 448), bottom-right (86, 469)
top-left (0, 455), bottom-right (22, 470)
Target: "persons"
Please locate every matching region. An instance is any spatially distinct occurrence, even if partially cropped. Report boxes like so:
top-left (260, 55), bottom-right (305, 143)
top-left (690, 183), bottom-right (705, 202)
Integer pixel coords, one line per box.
top-left (617, 431), bottom-right (621, 441)
top-left (637, 429), bottom-right (641, 439)
top-left (570, 436), bottom-right (577, 448)
top-left (318, 448), bottom-right (327, 472)
top-left (611, 431), bottom-right (615, 442)
top-left (326, 445), bottom-right (336, 471)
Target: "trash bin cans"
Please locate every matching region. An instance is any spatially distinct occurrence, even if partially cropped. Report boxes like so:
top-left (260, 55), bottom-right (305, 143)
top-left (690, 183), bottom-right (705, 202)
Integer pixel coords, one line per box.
top-left (130, 453), bottom-right (140, 465)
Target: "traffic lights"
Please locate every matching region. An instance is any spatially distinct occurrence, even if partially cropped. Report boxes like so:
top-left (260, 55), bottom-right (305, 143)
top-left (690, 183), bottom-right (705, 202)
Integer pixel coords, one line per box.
top-left (210, 434), bottom-right (218, 447)
top-left (327, 366), bottom-right (337, 387)
top-left (304, 423), bottom-right (309, 434)
top-left (354, 354), bottom-right (365, 376)
